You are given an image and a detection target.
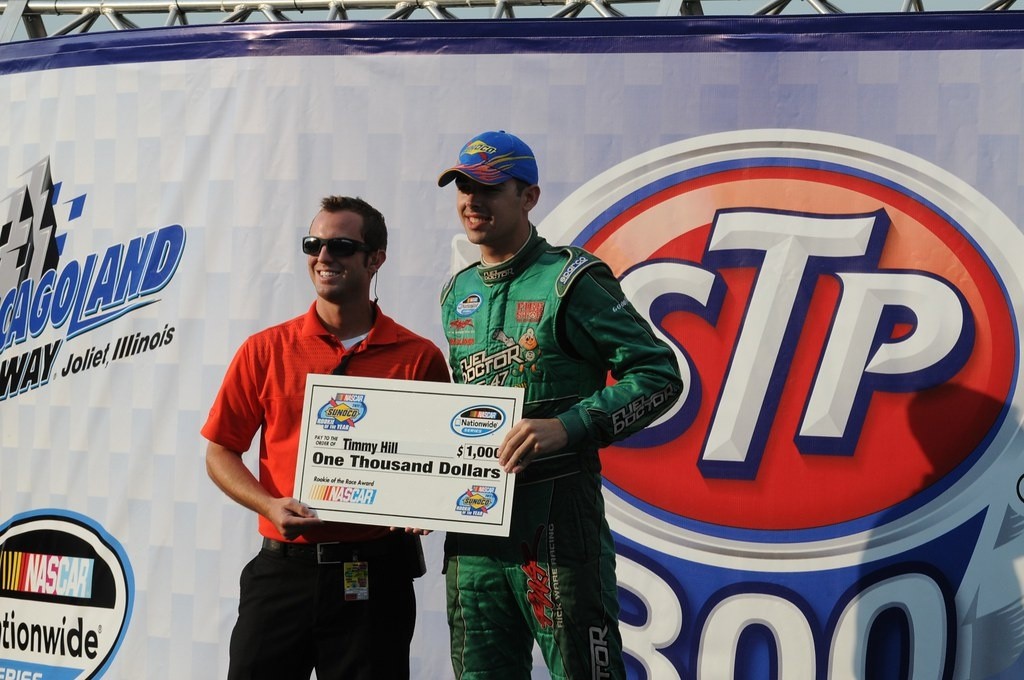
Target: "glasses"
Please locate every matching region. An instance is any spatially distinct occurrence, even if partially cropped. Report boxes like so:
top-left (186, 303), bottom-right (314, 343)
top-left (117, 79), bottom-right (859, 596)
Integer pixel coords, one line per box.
top-left (302, 236), bottom-right (371, 256)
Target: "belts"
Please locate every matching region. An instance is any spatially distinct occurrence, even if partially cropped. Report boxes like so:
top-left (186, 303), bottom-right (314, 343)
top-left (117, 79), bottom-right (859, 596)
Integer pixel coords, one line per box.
top-left (262, 537), bottom-right (408, 564)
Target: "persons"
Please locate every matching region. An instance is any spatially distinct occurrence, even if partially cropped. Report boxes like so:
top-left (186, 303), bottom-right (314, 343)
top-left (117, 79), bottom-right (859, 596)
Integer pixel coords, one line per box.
top-left (437, 131), bottom-right (683, 680)
top-left (201, 195), bottom-right (450, 680)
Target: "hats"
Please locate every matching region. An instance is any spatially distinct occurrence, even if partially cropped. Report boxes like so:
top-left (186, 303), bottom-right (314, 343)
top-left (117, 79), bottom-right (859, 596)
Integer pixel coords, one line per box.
top-left (438, 130), bottom-right (538, 185)
